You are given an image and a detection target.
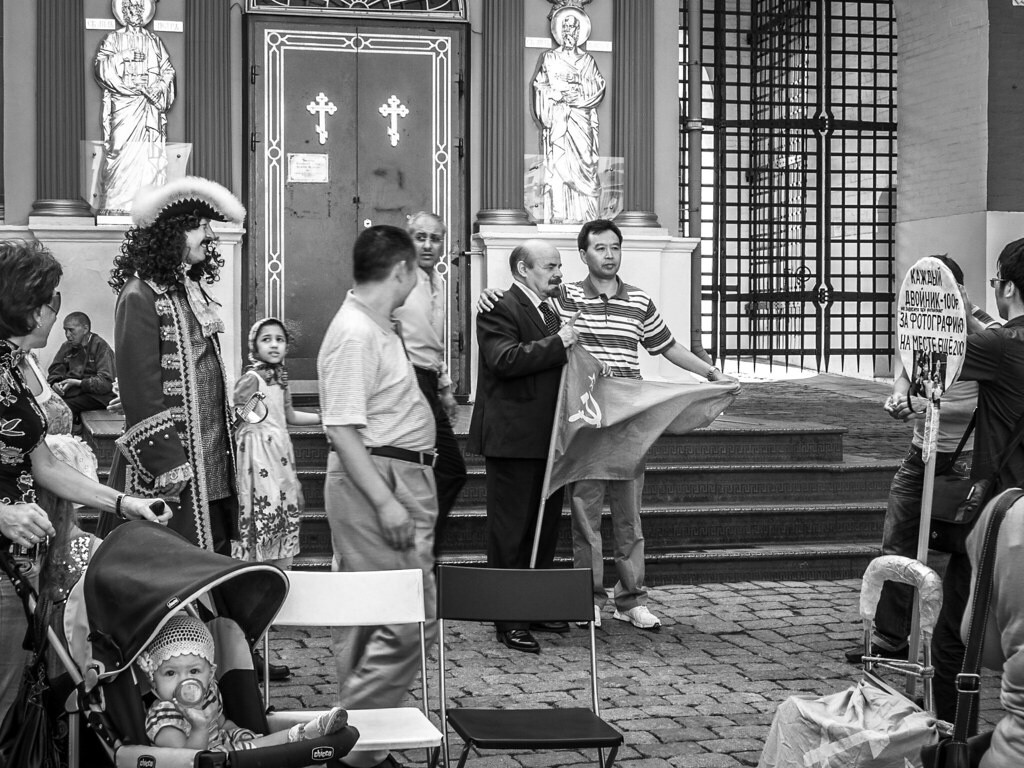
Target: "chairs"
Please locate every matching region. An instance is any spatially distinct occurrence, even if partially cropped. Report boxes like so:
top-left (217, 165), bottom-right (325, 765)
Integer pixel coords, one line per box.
top-left (262, 567), bottom-right (441, 768)
top-left (433, 563), bottom-right (624, 768)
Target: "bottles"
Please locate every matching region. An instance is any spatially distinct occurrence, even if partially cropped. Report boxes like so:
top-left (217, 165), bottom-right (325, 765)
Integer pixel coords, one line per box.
top-left (173, 678), bottom-right (205, 706)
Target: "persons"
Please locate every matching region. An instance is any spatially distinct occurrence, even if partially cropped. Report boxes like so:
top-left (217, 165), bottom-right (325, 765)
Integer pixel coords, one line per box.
top-left (0, 235), bottom-right (174, 768)
top-left (136, 614), bottom-right (348, 753)
top-left (389, 211), bottom-right (467, 572)
top-left (22, 347), bottom-right (76, 517)
top-left (92, 0), bottom-right (176, 216)
top-left (96, 175), bottom-right (290, 679)
top-left (230, 318), bottom-right (323, 570)
top-left (47, 311), bottom-right (116, 435)
top-left (477, 219), bottom-right (743, 629)
top-left (316, 226), bottom-right (442, 768)
top-left (533, 15), bottom-right (606, 224)
top-left (845, 251), bottom-right (1002, 662)
top-left (960, 487), bottom-right (1024, 767)
top-left (922, 237), bottom-right (1024, 739)
top-left (465, 236), bottom-right (582, 652)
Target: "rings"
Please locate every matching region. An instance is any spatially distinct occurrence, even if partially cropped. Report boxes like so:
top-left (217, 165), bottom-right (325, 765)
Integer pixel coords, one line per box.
top-left (27, 533), bottom-right (35, 540)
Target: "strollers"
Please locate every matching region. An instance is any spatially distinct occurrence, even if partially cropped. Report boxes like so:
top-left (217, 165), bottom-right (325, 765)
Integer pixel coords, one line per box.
top-left (1, 499), bottom-right (359, 768)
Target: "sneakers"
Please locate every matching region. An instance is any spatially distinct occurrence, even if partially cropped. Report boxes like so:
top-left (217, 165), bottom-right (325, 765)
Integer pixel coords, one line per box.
top-left (576, 604), bottom-right (602, 627)
top-left (613, 605), bottom-right (661, 628)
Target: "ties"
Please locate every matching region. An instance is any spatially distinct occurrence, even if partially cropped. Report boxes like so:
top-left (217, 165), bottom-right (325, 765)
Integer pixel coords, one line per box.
top-left (539, 302), bottom-right (559, 335)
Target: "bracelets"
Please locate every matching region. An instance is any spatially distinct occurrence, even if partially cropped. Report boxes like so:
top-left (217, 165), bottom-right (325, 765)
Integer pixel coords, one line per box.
top-left (707, 366), bottom-right (720, 381)
top-left (316, 414), bottom-right (322, 424)
top-left (115, 493), bottom-right (131, 519)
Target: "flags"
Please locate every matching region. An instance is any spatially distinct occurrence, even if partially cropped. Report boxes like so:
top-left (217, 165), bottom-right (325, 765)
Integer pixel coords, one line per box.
top-left (544, 340), bottom-right (739, 500)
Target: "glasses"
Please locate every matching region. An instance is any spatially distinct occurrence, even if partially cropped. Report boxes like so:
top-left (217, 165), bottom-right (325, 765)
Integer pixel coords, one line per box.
top-left (41, 292), bottom-right (61, 315)
top-left (412, 236), bottom-right (444, 246)
top-left (991, 278), bottom-right (1007, 288)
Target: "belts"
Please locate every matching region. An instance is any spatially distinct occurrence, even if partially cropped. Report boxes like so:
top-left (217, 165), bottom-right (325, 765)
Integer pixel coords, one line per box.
top-left (8, 536), bottom-right (49, 557)
top-left (371, 446), bottom-right (438, 468)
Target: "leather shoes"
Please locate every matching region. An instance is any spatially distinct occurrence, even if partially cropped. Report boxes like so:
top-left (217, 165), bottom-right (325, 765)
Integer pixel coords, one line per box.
top-left (529, 621), bottom-right (569, 632)
top-left (254, 656), bottom-right (290, 679)
top-left (497, 628), bottom-right (539, 652)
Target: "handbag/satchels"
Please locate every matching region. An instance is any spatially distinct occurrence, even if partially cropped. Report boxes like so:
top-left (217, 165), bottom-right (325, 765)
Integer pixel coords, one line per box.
top-left (0, 660), bottom-right (62, 768)
top-left (921, 730), bottom-right (993, 768)
top-left (930, 464), bottom-right (1002, 552)
top-left (45, 434), bottom-right (99, 507)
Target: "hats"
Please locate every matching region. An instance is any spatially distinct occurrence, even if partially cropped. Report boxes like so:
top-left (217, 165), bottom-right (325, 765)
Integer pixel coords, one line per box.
top-left (139, 615), bottom-right (217, 682)
top-left (131, 175), bottom-right (247, 229)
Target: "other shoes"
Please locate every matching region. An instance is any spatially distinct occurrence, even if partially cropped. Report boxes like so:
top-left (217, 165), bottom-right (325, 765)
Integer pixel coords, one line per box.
top-left (846, 640), bottom-right (909, 661)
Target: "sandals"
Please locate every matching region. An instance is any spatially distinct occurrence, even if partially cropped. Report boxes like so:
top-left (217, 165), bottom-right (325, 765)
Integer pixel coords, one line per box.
top-left (300, 707), bottom-right (348, 739)
